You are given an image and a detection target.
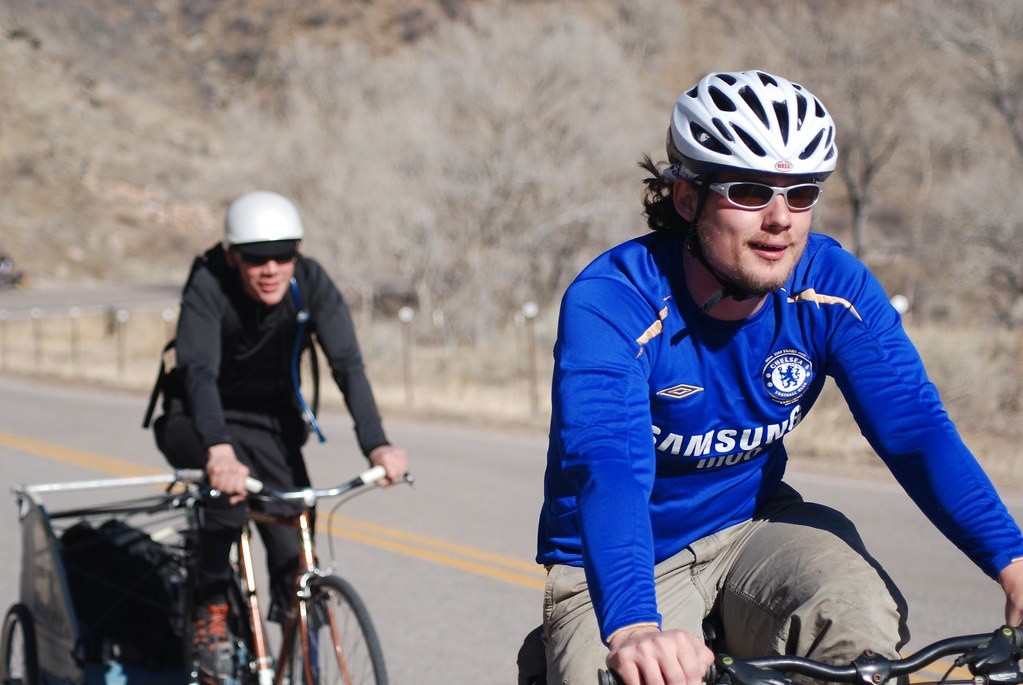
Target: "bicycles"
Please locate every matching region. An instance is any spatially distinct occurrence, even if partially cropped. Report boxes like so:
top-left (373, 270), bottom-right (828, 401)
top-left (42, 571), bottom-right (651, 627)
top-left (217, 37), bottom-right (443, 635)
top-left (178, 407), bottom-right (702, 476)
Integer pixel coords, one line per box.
top-left (513, 610), bottom-right (1023, 685)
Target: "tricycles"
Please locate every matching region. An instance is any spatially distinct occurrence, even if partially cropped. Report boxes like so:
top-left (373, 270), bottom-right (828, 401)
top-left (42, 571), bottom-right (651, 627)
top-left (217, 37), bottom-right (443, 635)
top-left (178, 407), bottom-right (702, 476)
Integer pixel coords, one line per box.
top-left (0, 459), bottom-right (418, 685)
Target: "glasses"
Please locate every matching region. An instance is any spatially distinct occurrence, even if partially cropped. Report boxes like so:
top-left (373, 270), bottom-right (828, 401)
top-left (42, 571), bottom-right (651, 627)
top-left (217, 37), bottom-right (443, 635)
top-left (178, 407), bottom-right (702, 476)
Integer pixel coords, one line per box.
top-left (236, 245), bottom-right (296, 265)
top-left (678, 166), bottom-right (822, 211)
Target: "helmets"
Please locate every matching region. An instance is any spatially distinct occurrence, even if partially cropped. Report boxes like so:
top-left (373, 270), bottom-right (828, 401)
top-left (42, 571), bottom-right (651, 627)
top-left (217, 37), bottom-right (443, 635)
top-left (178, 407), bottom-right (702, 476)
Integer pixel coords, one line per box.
top-left (223, 191), bottom-right (305, 251)
top-left (664, 71), bottom-right (838, 181)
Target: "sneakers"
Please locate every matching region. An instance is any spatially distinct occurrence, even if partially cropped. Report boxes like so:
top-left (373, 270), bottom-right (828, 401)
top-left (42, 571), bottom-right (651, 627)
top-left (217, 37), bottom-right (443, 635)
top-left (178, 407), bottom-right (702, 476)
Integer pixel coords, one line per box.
top-left (186, 601), bottom-right (234, 678)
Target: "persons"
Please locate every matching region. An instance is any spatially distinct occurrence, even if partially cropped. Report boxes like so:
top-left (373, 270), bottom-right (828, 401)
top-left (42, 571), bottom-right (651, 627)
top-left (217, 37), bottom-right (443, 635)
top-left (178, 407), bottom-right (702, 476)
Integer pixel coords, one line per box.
top-left (536, 71), bottom-right (1022, 684)
top-left (153, 192), bottom-right (408, 685)
